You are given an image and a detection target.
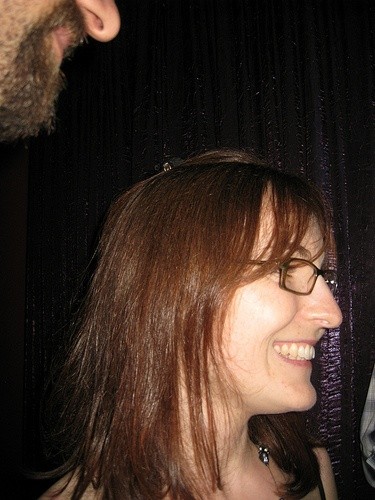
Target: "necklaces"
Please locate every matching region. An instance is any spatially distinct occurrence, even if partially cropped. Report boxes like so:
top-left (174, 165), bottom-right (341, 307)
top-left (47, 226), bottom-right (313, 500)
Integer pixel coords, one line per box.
top-left (257, 444), bottom-right (279, 490)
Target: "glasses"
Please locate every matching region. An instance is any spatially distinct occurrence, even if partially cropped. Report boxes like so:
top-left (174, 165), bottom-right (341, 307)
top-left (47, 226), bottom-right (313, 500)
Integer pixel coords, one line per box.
top-left (248, 258), bottom-right (339, 295)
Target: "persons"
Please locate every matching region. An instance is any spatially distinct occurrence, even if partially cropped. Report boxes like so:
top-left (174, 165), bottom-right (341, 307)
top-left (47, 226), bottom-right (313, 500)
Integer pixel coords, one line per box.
top-left (36, 146), bottom-right (343, 500)
top-left (359, 366), bottom-right (375, 488)
top-left (0, 0), bottom-right (122, 143)
